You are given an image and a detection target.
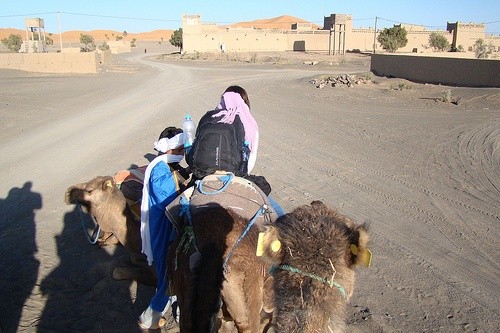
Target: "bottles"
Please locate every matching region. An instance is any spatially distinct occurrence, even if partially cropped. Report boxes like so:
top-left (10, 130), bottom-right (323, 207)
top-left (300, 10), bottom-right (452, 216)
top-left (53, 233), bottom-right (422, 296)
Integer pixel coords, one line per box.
top-left (183, 115), bottom-right (196, 147)
top-left (240, 141), bottom-right (250, 172)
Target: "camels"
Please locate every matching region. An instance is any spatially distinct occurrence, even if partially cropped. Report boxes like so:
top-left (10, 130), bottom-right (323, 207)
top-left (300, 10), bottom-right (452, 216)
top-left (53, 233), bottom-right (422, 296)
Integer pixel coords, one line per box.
top-left (64, 168), bottom-right (167, 282)
top-left (165, 170), bottom-right (374, 333)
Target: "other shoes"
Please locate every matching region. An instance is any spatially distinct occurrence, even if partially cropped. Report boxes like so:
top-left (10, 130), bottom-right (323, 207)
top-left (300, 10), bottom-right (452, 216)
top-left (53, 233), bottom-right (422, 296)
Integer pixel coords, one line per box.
top-left (137, 307), bottom-right (167, 329)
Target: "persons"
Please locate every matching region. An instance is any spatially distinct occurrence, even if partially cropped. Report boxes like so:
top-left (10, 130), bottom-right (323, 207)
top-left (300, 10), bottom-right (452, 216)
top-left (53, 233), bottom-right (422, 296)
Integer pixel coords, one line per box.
top-left (188, 86), bottom-right (284, 216)
top-left (136, 126), bottom-right (193, 330)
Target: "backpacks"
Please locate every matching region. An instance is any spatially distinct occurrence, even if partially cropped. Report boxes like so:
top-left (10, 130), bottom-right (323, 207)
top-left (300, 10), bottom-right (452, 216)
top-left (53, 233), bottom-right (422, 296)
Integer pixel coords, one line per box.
top-left (189, 109), bottom-right (248, 177)
